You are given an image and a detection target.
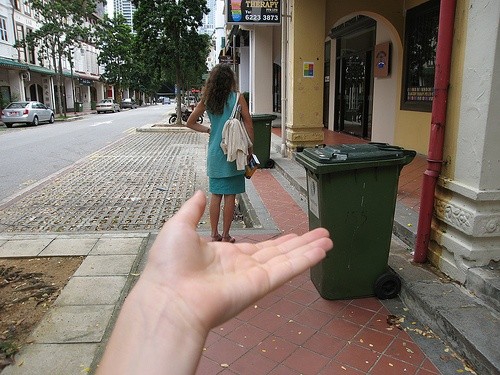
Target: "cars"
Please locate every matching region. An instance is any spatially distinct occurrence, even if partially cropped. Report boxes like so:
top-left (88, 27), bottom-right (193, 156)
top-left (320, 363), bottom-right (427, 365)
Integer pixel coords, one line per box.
top-left (119, 98), bottom-right (137, 109)
top-left (95, 98), bottom-right (121, 114)
top-left (157, 97), bottom-right (171, 104)
top-left (1, 101), bottom-right (55, 128)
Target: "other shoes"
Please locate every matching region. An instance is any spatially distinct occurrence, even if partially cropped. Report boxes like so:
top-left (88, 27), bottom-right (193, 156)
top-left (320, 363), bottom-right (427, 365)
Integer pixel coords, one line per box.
top-left (221, 235), bottom-right (235, 243)
top-left (209, 233), bottom-right (222, 242)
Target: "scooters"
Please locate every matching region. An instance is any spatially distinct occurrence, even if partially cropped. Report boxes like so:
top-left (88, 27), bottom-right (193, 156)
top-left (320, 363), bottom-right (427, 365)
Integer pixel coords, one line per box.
top-left (168, 107), bottom-right (204, 124)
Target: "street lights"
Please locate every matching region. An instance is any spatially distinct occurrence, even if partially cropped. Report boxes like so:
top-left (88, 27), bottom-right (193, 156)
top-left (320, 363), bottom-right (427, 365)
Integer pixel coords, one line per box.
top-left (68, 47), bottom-right (76, 114)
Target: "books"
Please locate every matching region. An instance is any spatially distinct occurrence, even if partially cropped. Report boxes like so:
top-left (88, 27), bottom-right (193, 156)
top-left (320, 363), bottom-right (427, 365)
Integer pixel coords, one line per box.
top-left (244, 153), bottom-right (260, 180)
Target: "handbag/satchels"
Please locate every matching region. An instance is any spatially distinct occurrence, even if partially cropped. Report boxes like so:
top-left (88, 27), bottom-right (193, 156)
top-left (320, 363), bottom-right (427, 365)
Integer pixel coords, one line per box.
top-left (221, 92), bottom-right (253, 171)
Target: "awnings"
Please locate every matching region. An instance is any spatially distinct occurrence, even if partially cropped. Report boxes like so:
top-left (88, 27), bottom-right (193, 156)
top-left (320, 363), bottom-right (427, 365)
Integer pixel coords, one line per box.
top-left (0, 58), bottom-right (100, 82)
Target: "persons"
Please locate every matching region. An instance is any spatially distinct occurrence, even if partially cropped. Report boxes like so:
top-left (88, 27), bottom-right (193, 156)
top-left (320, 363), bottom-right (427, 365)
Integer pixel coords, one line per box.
top-left (187, 62), bottom-right (255, 243)
top-left (95, 189), bottom-right (333, 374)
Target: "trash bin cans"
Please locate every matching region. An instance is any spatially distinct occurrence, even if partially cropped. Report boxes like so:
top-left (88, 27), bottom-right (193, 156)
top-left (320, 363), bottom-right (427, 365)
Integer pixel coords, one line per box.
top-left (248, 114), bottom-right (277, 169)
top-left (75, 101), bottom-right (83, 112)
top-left (294, 142), bottom-right (417, 300)
top-left (91, 101), bottom-right (97, 109)
